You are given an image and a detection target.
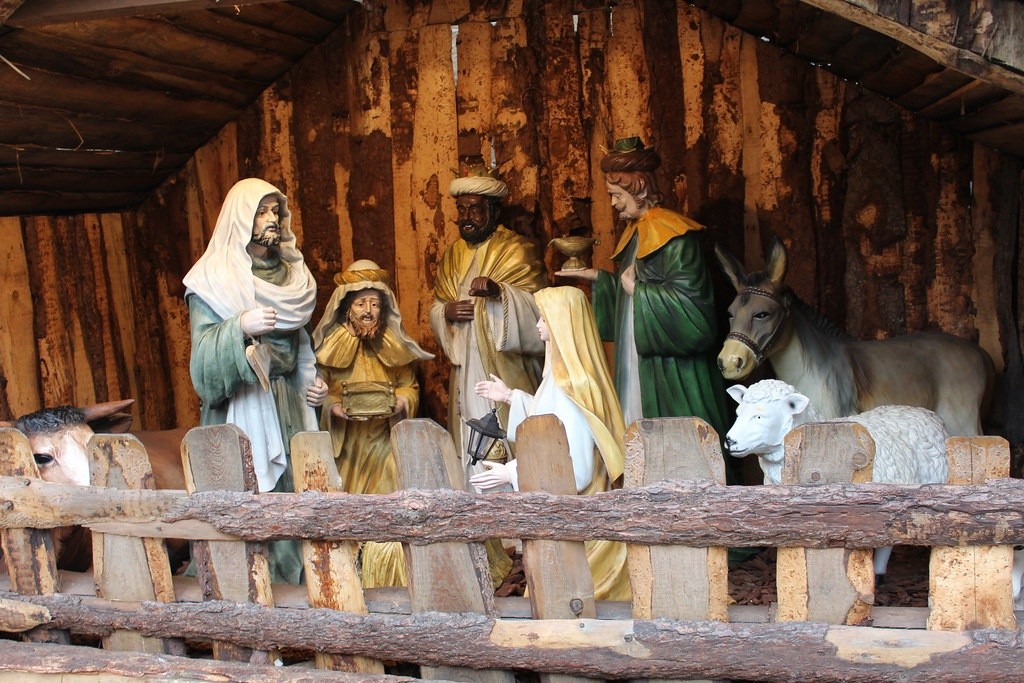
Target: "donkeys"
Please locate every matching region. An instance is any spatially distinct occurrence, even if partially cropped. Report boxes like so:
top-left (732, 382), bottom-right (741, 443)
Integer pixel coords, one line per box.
top-left (714, 241), bottom-right (999, 438)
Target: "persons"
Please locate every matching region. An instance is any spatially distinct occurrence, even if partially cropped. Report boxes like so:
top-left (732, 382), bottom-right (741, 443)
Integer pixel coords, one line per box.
top-left (470, 286), bottom-right (632, 600)
top-left (554, 138), bottom-right (763, 563)
top-left (182, 178), bottom-right (329, 586)
top-left (430, 178), bottom-right (551, 555)
top-left (311, 259), bottom-right (435, 493)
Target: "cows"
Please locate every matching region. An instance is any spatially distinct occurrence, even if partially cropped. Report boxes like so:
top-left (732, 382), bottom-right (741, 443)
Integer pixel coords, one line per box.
top-left (1, 399), bottom-right (192, 576)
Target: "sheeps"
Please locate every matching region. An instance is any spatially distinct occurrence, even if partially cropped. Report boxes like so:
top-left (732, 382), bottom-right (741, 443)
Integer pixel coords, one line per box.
top-left (723, 379), bottom-right (950, 575)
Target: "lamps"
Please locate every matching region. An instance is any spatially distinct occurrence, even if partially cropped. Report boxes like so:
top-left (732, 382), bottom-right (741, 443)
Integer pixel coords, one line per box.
top-left (466, 406), bottom-right (508, 467)
